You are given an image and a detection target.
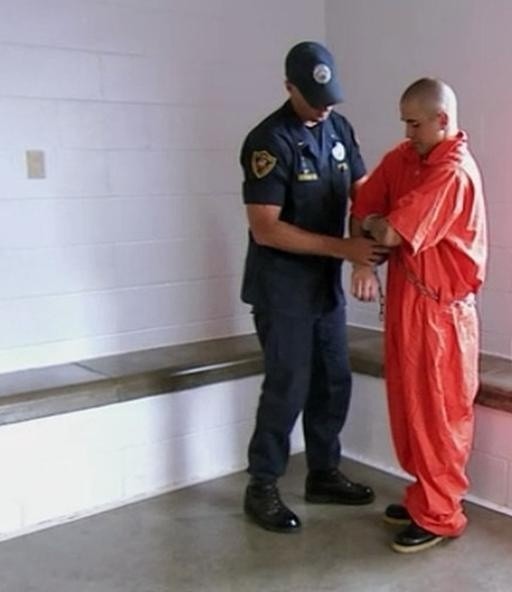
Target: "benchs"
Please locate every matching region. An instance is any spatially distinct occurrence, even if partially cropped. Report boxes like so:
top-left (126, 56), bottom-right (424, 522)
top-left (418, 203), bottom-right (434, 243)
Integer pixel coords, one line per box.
top-left (0, 332), bottom-right (309, 541)
top-left (339, 320), bottom-right (511, 514)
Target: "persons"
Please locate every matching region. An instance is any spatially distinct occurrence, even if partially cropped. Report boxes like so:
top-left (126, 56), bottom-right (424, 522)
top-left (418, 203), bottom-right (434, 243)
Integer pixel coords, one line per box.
top-left (240, 42), bottom-right (391, 533)
top-left (349, 78), bottom-right (486, 554)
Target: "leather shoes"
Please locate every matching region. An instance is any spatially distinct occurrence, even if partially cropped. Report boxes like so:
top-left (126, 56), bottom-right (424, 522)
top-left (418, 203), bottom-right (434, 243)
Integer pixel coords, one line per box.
top-left (389, 522), bottom-right (445, 554)
top-left (304, 465), bottom-right (376, 505)
top-left (382, 503), bottom-right (414, 525)
top-left (244, 478), bottom-right (303, 533)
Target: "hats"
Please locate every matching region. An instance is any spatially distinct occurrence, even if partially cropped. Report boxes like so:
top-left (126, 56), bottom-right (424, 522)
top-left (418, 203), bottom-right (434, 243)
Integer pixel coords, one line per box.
top-left (283, 40), bottom-right (346, 109)
